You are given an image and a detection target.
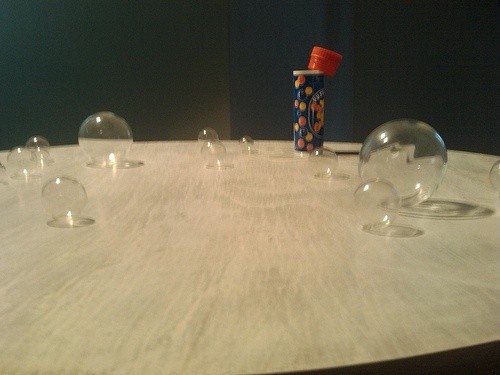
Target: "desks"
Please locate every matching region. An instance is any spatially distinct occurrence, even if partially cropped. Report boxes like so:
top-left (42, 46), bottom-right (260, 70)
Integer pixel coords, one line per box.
top-left (0, 139), bottom-right (499, 375)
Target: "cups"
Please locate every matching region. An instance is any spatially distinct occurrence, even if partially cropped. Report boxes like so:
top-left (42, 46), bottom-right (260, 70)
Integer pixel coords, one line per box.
top-left (292, 67), bottom-right (326, 156)
